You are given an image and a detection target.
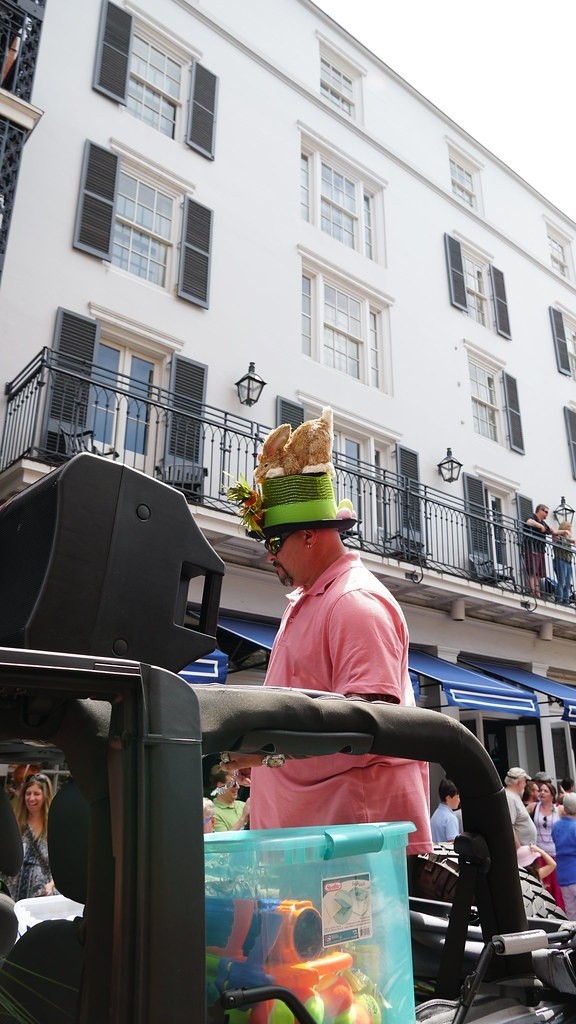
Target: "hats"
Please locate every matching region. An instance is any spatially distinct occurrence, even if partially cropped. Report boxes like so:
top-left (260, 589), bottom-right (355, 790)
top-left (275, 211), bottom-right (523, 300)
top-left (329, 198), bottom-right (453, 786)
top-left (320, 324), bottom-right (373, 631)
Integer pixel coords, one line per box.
top-left (220, 472), bottom-right (358, 539)
top-left (517, 845), bottom-right (542, 868)
top-left (507, 767), bottom-right (532, 781)
top-left (535, 771), bottom-right (552, 781)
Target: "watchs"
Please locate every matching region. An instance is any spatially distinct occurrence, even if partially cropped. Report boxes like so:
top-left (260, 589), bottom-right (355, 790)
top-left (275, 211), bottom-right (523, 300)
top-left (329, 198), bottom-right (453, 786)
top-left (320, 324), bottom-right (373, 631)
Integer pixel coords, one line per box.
top-left (262, 754), bottom-right (285, 769)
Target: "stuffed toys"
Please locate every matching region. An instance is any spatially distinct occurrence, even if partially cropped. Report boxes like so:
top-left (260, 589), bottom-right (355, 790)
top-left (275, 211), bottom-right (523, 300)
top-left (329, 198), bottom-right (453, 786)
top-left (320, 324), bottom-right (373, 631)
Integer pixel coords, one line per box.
top-left (252, 406), bottom-right (336, 477)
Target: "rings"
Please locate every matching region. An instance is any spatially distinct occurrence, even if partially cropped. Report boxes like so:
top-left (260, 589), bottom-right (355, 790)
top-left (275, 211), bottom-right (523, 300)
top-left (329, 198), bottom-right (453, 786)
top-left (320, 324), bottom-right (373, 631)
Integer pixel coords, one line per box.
top-left (220, 753), bottom-right (230, 763)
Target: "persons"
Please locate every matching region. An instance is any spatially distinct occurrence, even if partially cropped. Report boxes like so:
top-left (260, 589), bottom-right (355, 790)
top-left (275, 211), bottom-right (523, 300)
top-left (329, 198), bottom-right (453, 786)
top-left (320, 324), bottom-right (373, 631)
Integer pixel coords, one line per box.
top-left (523, 504), bottom-right (572, 597)
top-left (552, 520), bottom-right (576, 607)
top-left (219, 473), bottom-right (435, 857)
top-left (429, 767), bottom-right (576, 921)
top-left (0, 764), bottom-right (64, 903)
top-left (203, 761), bottom-right (254, 868)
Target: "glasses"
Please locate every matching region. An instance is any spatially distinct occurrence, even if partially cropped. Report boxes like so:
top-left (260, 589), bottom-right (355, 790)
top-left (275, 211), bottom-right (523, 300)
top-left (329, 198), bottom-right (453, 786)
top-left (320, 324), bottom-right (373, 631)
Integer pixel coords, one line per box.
top-left (541, 509), bottom-right (549, 514)
top-left (263, 531), bottom-right (297, 555)
top-left (534, 789), bottom-right (540, 792)
top-left (543, 816), bottom-right (548, 829)
top-left (26, 773), bottom-right (47, 785)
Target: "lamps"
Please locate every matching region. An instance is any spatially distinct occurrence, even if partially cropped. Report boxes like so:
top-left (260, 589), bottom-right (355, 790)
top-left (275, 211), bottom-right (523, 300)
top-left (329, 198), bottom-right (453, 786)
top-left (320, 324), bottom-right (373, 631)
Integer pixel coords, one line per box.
top-left (540, 622), bottom-right (552, 641)
top-left (552, 497), bottom-right (575, 525)
top-left (233, 361), bottom-right (267, 407)
top-left (438, 448), bottom-right (462, 483)
top-left (450, 599), bottom-right (466, 620)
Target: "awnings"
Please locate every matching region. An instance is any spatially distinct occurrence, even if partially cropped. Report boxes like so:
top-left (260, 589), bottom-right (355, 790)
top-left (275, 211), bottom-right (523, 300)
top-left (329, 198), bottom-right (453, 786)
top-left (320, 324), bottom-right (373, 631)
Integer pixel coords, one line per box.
top-left (406, 648), bottom-right (541, 719)
top-left (457, 656), bottom-right (576, 722)
top-left (190, 609), bottom-right (420, 700)
top-left (177, 647), bottom-right (230, 684)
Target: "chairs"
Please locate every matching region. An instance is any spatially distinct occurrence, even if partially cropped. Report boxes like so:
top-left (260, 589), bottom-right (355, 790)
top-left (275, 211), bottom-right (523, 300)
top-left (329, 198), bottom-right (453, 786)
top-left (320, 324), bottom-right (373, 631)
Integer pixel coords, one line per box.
top-left (381, 531), bottom-right (426, 566)
top-left (469, 554), bottom-right (517, 592)
top-left (0, 773), bottom-right (113, 1024)
top-left (58, 422), bottom-right (119, 461)
top-left (545, 578), bottom-right (576, 607)
top-left (155, 463), bottom-right (208, 503)
top-left (341, 530), bottom-right (363, 548)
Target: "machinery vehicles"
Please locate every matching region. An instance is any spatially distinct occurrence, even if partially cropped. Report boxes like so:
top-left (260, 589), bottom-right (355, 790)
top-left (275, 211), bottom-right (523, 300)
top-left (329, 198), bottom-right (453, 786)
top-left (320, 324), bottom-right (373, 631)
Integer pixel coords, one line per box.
top-left (0, 452), bottom-right (576, 1024)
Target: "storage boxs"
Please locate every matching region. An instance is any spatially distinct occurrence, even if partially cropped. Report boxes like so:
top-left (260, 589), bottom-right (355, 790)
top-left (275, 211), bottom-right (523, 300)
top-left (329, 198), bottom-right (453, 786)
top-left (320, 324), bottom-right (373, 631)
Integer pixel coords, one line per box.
top-left (204, 820), bottom-right (417, 1024)
